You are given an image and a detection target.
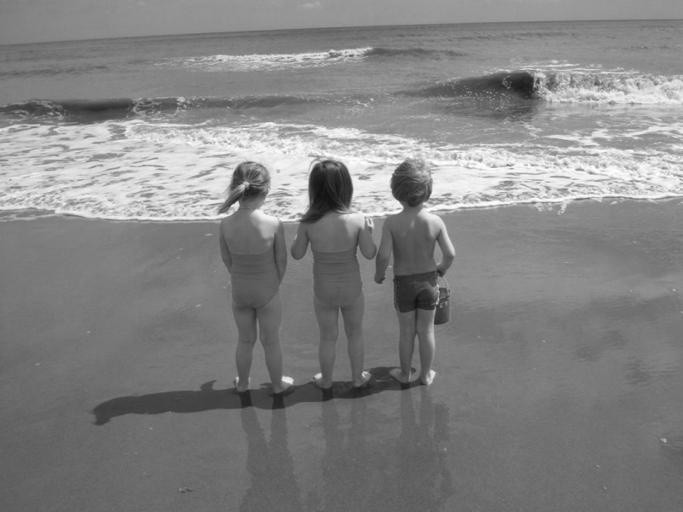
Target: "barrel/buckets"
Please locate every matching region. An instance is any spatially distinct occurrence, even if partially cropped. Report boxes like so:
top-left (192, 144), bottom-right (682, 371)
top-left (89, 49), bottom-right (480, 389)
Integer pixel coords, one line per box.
top-left (433, 271), bottom-right (451, 325)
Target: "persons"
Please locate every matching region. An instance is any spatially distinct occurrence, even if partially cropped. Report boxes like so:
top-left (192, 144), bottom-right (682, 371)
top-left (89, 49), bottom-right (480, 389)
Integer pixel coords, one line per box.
top-left (375, 159), bottom-right (455, 388)
top-left (216, 162), bottom-right (295, 394)
top-left (289, 156), bottom-right (377, 392)
